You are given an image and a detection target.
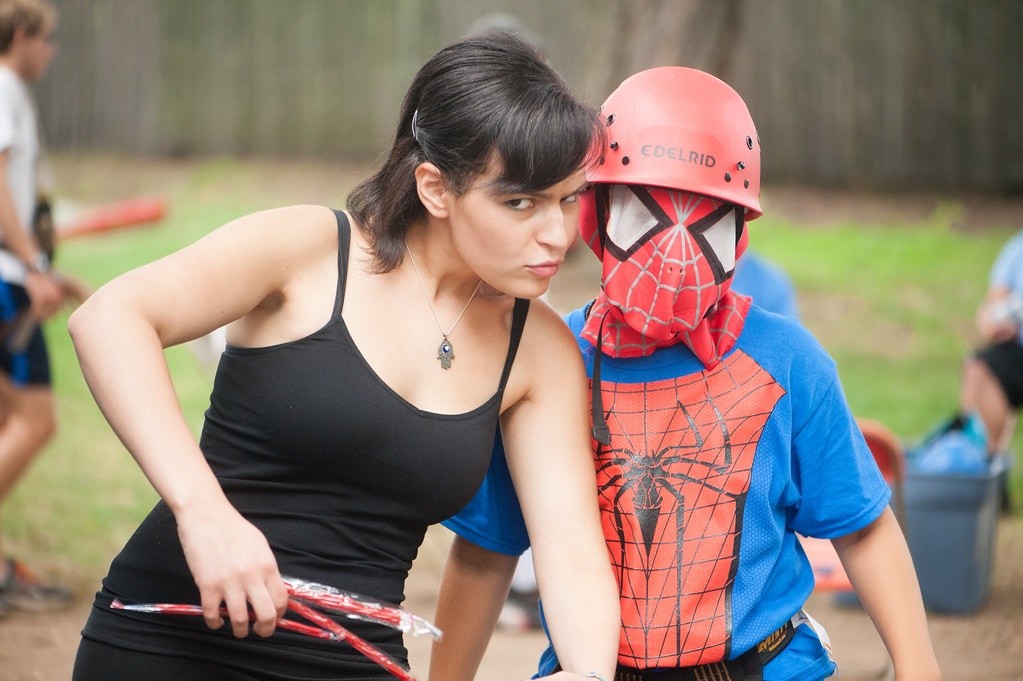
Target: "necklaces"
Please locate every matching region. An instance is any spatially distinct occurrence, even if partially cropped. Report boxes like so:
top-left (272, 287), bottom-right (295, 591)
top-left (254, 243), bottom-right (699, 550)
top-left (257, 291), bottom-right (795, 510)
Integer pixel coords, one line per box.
top-left (400, 230), bottom-right (483, 370)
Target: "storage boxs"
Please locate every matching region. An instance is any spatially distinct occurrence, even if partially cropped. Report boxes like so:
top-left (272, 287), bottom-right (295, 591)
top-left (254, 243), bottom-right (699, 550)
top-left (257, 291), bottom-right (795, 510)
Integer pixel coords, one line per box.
top-left (836, 455), bottom-right (1010, 613)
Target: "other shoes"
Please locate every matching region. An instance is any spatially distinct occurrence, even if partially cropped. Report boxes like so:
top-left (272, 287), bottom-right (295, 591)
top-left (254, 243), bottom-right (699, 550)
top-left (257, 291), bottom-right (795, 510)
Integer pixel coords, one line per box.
top-left (0, 558), bottom-right (76, 614)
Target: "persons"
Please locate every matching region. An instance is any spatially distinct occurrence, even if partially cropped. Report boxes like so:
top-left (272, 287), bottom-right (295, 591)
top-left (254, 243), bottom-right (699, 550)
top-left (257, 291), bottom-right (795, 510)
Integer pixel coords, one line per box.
top-left (71, 29), bottom-right (622, 681)
top-left (427, 66), bottom-right (944, 681)
top-left (960, 230), bottom-right (1023, 514)
top-left (0, 0), bottom-right (101, 617)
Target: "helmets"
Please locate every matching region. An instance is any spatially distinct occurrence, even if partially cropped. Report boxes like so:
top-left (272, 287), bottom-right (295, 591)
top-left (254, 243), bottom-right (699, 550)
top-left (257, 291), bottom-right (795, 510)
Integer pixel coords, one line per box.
top-left (576, 66), bottom-right (764, 221)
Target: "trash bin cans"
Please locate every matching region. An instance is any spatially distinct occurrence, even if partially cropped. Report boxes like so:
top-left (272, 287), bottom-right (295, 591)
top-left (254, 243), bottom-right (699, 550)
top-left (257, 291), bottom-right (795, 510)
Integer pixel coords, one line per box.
top-left (834, 448), bottom-right (1011, 617)
